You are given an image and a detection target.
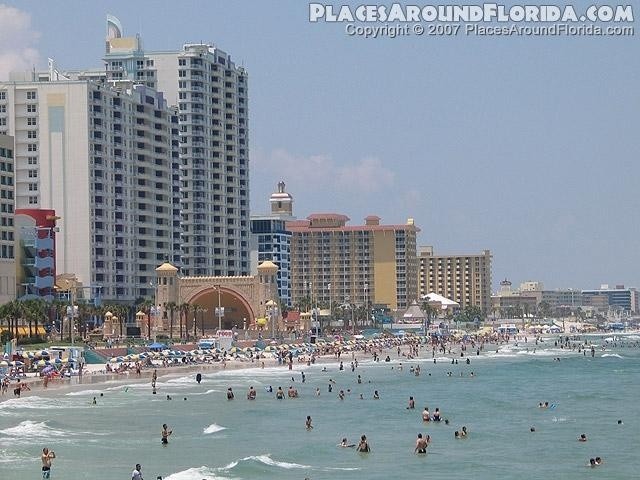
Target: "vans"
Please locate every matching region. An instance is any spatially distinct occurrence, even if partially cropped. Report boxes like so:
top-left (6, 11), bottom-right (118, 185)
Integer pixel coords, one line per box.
top-left (398, 318), bottom-right (405, 324)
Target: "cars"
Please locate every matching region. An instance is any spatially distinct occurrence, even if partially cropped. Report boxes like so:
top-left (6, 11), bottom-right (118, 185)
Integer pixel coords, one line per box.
top-left (148, 342), bottom-right (169, 352)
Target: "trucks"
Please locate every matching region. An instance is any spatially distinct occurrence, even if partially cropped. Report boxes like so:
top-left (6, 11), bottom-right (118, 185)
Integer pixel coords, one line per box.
top-left (197, 338), bottom-right (217, 350)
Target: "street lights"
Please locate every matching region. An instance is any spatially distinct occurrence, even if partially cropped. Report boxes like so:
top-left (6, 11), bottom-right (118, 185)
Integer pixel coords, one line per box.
top-left (568, 288), bottom-right (575, 306)
top-left (303, 280), bottom-right (370, 326)
top-left (561, 304), bottom-right (570, 331)
top-left (72, 284), bottom-right (103, 345)
top-left (381, 308), bottom-right (385, 314)
top-left (213, 283), bottom-right (223, 328)
